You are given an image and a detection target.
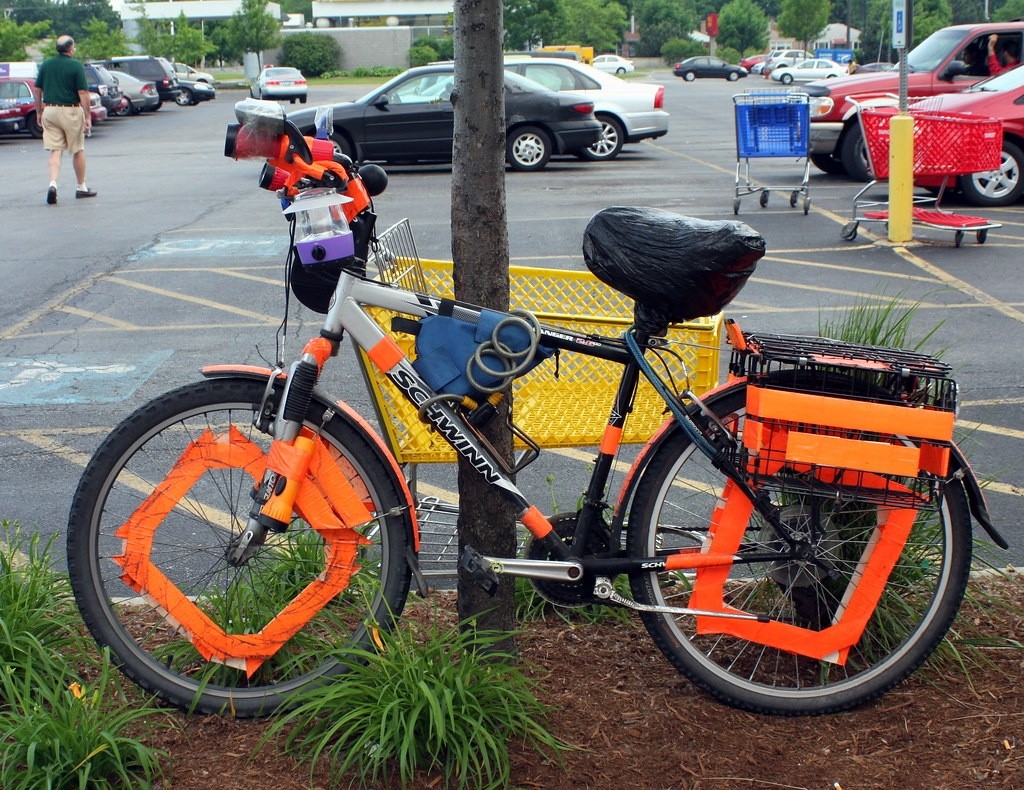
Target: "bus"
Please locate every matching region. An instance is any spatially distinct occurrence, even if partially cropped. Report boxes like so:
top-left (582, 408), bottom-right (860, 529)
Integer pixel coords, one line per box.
top-left (816, 49), bottom-right (856, 63)
top-left (537, 45), bottom-right (594, 68)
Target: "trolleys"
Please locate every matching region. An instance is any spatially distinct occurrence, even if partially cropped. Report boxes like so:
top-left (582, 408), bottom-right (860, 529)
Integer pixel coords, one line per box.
top-left (729, 92), bottom-right (812, 215)
top-left (842, 92), bottom-right (1004, 248)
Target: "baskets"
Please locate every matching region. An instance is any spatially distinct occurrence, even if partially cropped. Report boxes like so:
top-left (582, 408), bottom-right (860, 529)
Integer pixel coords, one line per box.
top-left (727, 332), bottom-right (958, 511)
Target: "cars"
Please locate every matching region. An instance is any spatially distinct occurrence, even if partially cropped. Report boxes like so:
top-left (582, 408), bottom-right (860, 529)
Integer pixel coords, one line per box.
top-left (593, 54), bottom-right (635, 76)
top-left (672, 55), bottom-right (750, 82)
top-left (287, 63), bottom-right (605, 175)
top-left (910, 63), bottom-right (1024, 206)
top-left (0, 61), bottom-right (160, 140)
top-left (431, 55), bottom-right (670, 160)
top-left (171, 63), bottom-right (216, 106)
top-left (250, 67), bottom-right (308, 104)
top-left (853, 62), bottom-right (895, 73)
top-left (771, 58), bottom-right (851, 84)
top-left (740, 54), bottom-right (769, 75)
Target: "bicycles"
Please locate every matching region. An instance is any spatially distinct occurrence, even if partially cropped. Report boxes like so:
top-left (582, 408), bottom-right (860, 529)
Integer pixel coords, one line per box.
top-left (66, 91), bottom-right (1010, 717)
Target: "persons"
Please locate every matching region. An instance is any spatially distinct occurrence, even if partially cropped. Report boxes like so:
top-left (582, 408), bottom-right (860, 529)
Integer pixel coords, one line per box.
top-left (984, 34), bottom-right (1020, 77)
top-left (34, 35), bottom-right (97, 205)
top-left (846, 60), bottom-right (856, 75)
top-left (960, 42), bottom-right (990, 77)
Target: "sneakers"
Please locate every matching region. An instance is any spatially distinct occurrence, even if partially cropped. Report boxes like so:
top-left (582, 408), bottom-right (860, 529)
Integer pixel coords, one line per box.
top-left (76, 189), bottom-right (97, 198)
top-left (47, 186), bottom-right (57, 203)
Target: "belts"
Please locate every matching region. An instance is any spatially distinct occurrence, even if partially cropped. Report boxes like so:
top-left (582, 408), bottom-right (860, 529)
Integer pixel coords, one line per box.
top-left (45, 103), bottom-right (79, 107)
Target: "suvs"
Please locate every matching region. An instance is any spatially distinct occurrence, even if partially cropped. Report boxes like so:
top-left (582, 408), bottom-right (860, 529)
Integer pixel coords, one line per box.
top-left (789, 21), bottom-right (1024, 181)
top-left (765, 50), bottom-right (814, 70)
top-left (89, 54), bottom-right (179, 112)
